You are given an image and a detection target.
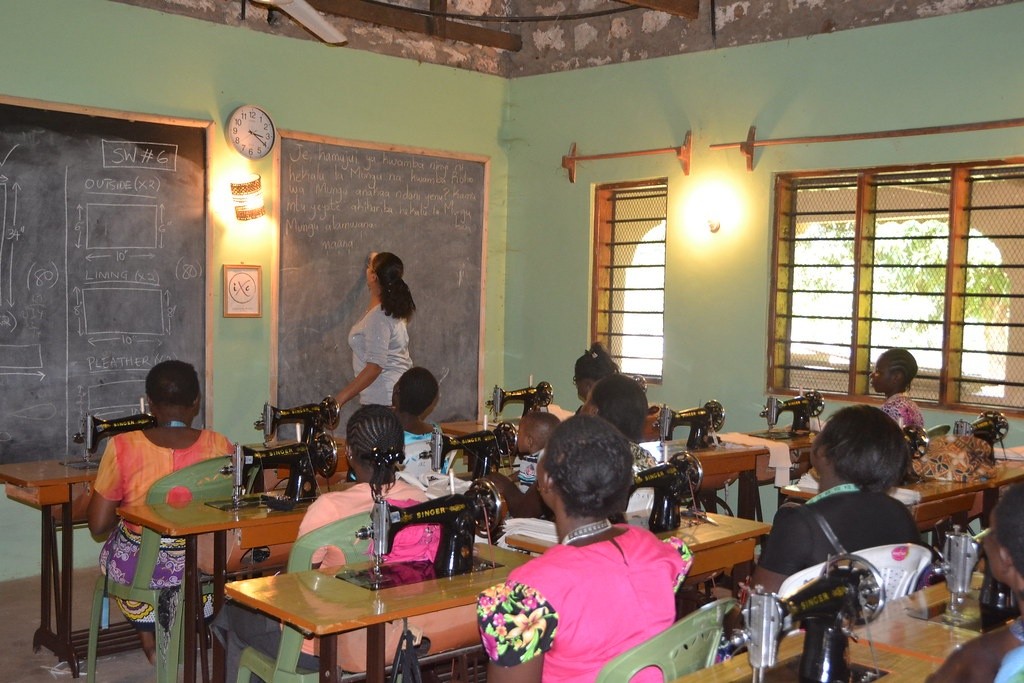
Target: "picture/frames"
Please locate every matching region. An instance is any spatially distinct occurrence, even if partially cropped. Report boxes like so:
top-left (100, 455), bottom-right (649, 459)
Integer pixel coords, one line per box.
top-left (223, 263), bottom-right (264, 319)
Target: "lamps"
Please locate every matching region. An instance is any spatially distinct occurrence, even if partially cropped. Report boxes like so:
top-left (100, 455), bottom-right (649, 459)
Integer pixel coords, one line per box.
top-left (230, 174), bottom-right (267, 220)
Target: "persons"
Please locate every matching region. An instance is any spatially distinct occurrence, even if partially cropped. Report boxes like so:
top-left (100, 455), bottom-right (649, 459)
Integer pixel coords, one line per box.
top-left (869, 350), bottom-right (922, 430)
top-left (573, 342), bottom-right (621, 402)
top-left (85, 361), bottom-right (236, 664)
top-left (922, 482), bottom-right (1024, 683)
top-left (336, 252), bottom-right (416, 408)
top-left (508, 412), bottom-right (560, 494)
top-left (211, 405), bottom-right (429, 683)
top-left (392, 367), bottom-right (441, 443)
top-left (477, 414), bottom-right (695, 683)
top-left (484, 374), bottom-right (655, 520)
top-left (752, 406), bottom-right (920, 595)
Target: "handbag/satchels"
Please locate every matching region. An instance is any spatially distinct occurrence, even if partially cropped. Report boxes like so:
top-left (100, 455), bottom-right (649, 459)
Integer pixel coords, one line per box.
top-left (912, 434), bottom-right (998, 483)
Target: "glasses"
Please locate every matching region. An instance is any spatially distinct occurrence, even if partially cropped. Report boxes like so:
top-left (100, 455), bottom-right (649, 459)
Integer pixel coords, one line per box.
top-left (367, 263), bottom-right (378, 280)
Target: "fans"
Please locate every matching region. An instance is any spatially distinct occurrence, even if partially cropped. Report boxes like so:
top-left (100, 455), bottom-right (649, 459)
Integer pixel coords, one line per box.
top-left (253, 0), bottom-right (349, 43)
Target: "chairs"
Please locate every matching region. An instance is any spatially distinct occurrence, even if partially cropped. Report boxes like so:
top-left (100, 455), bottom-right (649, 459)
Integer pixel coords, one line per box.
top-left (237, 512), bottom-right (403, 683)
top-left (926, 425), bottom-right (951, 438)
top-left (87, 456), bottom-right (260, 683)
top-left (596, 597), bottom-right (735, 683)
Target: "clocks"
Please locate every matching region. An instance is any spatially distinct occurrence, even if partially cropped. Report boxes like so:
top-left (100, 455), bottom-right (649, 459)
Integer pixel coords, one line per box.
top-left (227, 105), bottom-right (276, 160)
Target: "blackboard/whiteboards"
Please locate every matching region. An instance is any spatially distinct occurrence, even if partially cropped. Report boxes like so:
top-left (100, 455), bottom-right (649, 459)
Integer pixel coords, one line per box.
top-left (0, 94), bottom-right (213, 464)
top-left (271, 128), bottom-right (491, 440)
